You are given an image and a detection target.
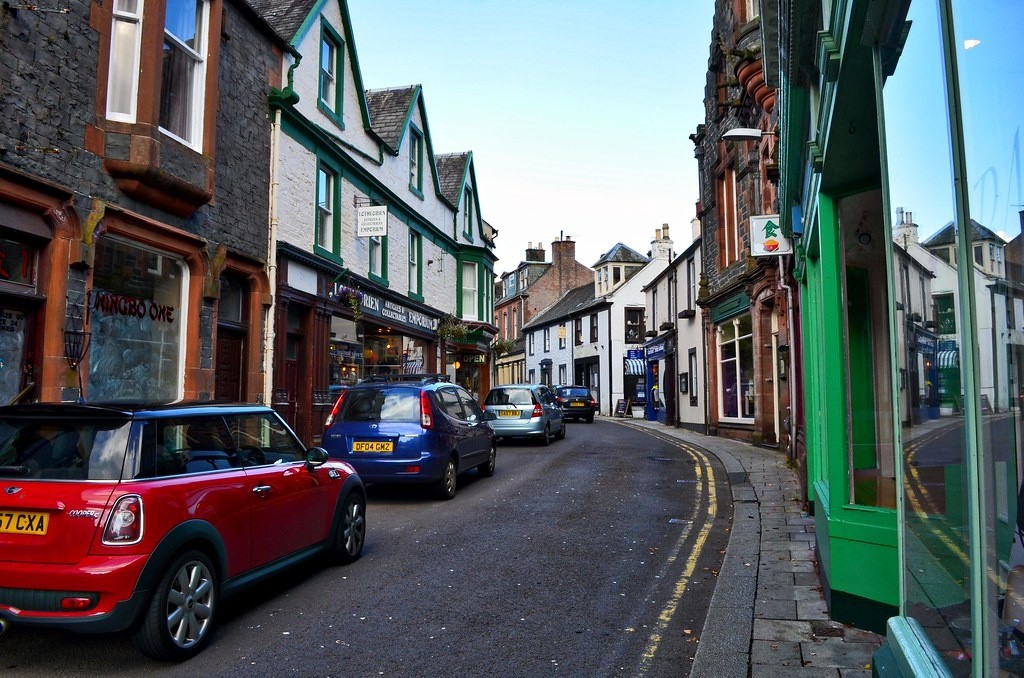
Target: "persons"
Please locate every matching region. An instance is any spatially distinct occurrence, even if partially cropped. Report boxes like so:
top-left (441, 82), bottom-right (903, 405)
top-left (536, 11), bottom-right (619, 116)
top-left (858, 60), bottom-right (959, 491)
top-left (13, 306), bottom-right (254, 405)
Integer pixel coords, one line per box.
top-left (186, 425), bottom-right (216, 449)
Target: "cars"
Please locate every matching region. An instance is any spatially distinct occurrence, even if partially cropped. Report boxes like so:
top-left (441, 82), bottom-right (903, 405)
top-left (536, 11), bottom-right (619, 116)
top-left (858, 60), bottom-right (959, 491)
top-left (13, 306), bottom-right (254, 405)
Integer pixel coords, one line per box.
top-left (0, 400), bottom-right (367, 667)
top-left (480, 384), bottom-right (567, 451)
top-left (548, 383), bottom-right (595, 427)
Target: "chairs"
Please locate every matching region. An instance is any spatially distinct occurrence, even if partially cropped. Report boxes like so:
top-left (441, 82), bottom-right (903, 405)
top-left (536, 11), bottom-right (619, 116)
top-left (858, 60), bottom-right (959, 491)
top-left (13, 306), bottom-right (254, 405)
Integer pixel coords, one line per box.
top-left (178, 422), bottom-right (234, 472)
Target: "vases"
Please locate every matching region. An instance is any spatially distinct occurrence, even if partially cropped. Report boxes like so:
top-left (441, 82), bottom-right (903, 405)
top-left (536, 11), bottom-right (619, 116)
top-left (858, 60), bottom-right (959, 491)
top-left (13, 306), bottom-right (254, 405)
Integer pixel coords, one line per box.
top-left (339, 297), bottom-right (361, 307)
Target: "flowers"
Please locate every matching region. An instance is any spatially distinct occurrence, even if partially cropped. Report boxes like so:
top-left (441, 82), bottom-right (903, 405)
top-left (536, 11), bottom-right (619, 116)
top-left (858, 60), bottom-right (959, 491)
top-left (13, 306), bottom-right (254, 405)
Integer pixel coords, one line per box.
top-left (338, 286), bottom-right (364, 327)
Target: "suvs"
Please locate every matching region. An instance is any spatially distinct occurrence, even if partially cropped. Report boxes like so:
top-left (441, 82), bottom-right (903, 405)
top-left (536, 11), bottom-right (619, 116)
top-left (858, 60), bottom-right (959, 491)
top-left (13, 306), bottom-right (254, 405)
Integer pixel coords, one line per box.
top-left (321, 372), bottom-right (497, 502)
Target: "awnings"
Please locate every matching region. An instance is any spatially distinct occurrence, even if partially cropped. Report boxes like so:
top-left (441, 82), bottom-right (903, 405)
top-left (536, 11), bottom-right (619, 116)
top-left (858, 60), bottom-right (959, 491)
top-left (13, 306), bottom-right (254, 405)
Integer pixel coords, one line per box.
top-left (403, 362), bottom-right (423, 374)
top-left (625, 358), bottom-right (647, 375)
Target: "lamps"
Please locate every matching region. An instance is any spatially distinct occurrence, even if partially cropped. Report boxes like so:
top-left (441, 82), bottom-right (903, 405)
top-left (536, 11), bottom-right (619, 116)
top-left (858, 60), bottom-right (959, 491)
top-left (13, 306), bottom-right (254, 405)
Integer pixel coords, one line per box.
top-left (721, 127), bottom-right (775, 141)
